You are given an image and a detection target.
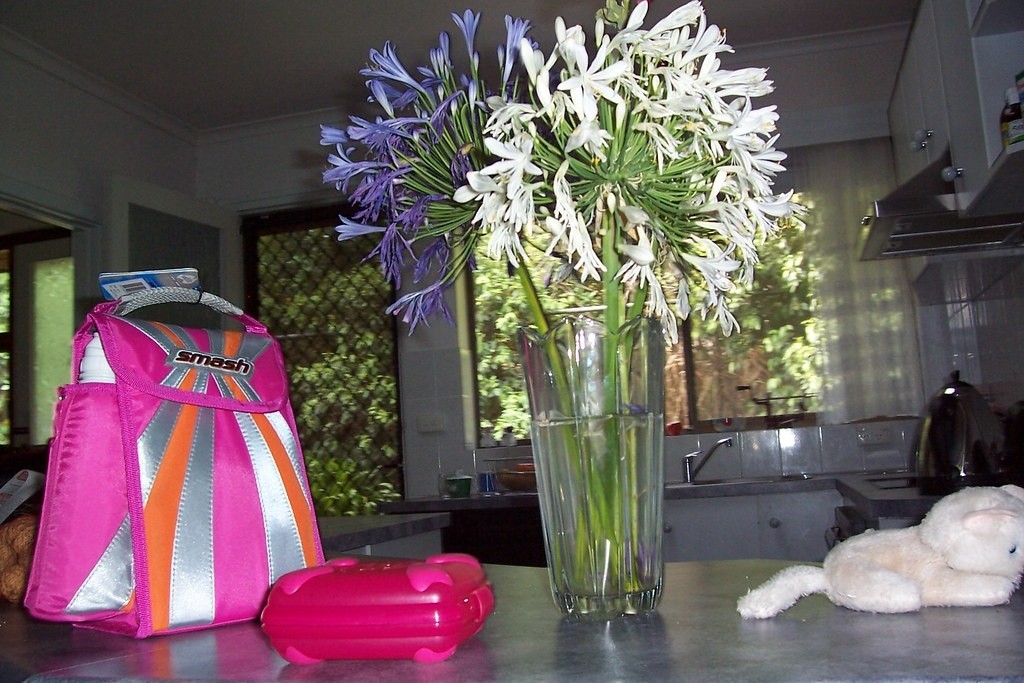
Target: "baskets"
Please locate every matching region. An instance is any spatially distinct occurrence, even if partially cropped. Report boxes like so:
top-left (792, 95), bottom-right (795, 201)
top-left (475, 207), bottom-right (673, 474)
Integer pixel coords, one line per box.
top-left (497, 471), bottom-right (535, 491)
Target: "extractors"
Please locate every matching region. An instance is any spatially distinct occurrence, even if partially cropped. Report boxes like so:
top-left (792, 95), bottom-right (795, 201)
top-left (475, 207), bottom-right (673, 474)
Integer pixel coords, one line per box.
top-left (852, 193), bottom-right (1024, 260)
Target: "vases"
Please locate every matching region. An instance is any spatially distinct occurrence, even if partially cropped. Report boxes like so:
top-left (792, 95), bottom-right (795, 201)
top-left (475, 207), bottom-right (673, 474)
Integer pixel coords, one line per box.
top-left (516, 313), bottom-right (666, 622)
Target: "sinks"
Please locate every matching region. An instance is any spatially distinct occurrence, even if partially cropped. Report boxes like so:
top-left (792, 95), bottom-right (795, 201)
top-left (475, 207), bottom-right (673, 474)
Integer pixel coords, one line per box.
top-left (691, 475), bottom-right (814, 486)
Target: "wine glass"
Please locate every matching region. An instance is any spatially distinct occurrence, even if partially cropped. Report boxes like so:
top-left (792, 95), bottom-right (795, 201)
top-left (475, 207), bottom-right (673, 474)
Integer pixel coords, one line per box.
top-left (897, 431), bottom-right (912, 472)
top-left (855, 422), bottom-right (874, 475)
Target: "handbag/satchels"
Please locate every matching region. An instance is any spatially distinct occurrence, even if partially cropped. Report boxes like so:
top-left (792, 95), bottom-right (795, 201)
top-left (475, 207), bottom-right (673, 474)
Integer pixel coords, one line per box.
top-left (23, 287), bottom-right (326, 639)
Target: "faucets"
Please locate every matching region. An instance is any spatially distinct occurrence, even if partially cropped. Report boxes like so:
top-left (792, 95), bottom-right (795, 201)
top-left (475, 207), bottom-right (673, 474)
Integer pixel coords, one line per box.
top-left (681, 437), bottom-right (733, 484)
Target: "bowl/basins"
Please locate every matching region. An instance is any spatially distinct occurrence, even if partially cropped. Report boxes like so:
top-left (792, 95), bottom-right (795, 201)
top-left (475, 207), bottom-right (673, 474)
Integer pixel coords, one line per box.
top-left (497, 470), bottom-right (535, 492)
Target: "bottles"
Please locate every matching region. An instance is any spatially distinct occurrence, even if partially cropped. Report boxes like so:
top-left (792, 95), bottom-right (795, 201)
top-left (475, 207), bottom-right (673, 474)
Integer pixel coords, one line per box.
top-left (1006, 94), bottom-right (1024, 144)
top-left (1000, 87), bottom-right (1017, 148)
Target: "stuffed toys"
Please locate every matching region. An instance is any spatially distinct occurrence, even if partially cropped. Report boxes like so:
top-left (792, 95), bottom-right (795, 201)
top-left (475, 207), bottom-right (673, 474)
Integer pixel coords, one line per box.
top-left (736, 485), bottom-right (1024, 618)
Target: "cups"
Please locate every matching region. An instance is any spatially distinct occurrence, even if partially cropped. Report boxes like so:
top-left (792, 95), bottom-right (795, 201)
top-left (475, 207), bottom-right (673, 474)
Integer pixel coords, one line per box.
top-left (446, 477), bottom-right (471, 498)
top-left (464, 442), bottom-right (486, 495)
top-left (479, 471), bottom-right (496, 497)
top-left (665, 421), bottom-right (682, 436)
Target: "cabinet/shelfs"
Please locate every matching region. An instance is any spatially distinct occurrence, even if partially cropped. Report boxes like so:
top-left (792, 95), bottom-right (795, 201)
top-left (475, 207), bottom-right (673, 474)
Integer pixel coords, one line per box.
top-left (670, 490), bottom-right (917, 561)
top-left (850, 0), bottom-right (1024, 263)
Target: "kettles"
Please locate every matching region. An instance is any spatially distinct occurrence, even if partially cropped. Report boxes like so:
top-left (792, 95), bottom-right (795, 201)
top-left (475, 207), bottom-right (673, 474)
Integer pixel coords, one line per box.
top-left (916, 371), bottom-right (1009, 493)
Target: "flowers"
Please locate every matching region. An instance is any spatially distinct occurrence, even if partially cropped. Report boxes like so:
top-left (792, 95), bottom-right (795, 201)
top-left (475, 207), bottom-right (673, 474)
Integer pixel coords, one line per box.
top-left (318, 0), bottom-right (807, 348)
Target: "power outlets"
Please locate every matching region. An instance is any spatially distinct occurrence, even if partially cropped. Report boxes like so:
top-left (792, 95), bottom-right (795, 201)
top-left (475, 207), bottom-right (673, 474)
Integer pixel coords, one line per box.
top-left (857, 428), bottom-right (893, 447)
